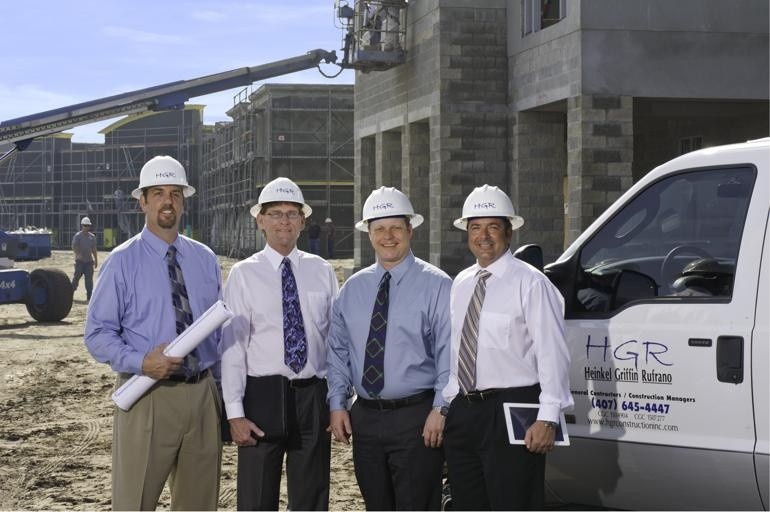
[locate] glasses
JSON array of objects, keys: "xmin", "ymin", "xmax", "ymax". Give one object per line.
[{"xmin": 264, "ymin": 211, "xmax": 300, "ymax": 219}]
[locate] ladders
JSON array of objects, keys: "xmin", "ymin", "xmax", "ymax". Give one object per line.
[
  {"xmin": 4, "ymin": 155, "xmax": 17, "ymax": 182},
  {"xmin": 122, "ymin": 146, "xmax": 136, "ymax": 179}
]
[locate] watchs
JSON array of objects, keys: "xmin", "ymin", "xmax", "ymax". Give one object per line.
[
  {"xmin": 435, "ymin": 404, "xmax": 450, "ymax": 419},
  {"xmin": 543, "ymin": 420, "xmax": 559, "ymax": 429}
]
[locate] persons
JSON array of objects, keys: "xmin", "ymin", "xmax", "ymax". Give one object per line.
[
  {"xmin": 324, "ymin": 186, "xmax": 454, "ymax": 510},
  {"xmin": 442, "ymin": 183, "xmax": 577, "ymax": 510},
  {"xmin": 325, "ymin": 218, "xmax": 336, "ymax": 258},
  {"xmin": 71, "ymin": 216, "xmax": 99, "ymax": 300},
  {"xmin": 219, "ymin": 175, "xmax": 352, "ymax": 510},
  {"xmin": 308, "ymin": 219, "xmax": 322, "ymax": 256},
  {"xmin": 83, "ymin": 154, "xmax": 232, "ymax": 510}
]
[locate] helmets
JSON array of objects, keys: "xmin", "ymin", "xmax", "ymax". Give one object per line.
[
  {"xmin": 250, "ymin": 177, "xmax": 312, "ymax": 219},
  {"xmin": 132, "ymin": 156, "xmax": 196, "ymax": 200},
  {"xmin": 453, "ymin": 184, "xmax": 524, "ymax": 231},
  {"xmin": 355, "ymin": 186, "xmax": 424, "ymax": 232},
  {"xmin": 81, "ymin": 217, "xmax": 92, "ymax": 225}
]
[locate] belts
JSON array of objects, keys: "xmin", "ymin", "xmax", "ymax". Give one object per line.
[
  {"xmin": 168, "ymin": 368, "xmax": 208, "ymax": 384},
  {"xmin": 289, "ymin": 377, "xmax": 319, "ymax": 389},
  {"xmin": 459, "ymin": 384, "xmax": 540, "ymax": 401},
  {"xmin": 357, "ymin": 390, "xmax": 434, "ymax": 409}
]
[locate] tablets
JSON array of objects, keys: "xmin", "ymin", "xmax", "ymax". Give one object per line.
[{"xmin": 503, "ymin": 402, "xmax": 571, "ymax": 446}]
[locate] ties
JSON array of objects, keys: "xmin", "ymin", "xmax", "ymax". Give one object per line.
[
  {"xmin": 282, "ymin": 257, "xmax": 307, "ymax": 374},
  {"xmin": 458, "ymin": 270, "xmax": 492, "ymax": 397},
  {"xmin": 361, "ymin": 273, "xmax": 389, "ymax": 397},
  {"xmin": 166, "ymin": 246, "xmax": 201, "ymax": 377}
]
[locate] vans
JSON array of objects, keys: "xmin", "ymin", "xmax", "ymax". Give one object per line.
[{"xmin": 513, "ymin": 138, "xmax": 769, "ymax": 507}]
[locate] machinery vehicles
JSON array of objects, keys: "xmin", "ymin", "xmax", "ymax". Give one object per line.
[{"xmin": 2, "ymin": 1, "xmax": 409, "ymax": 321}]
